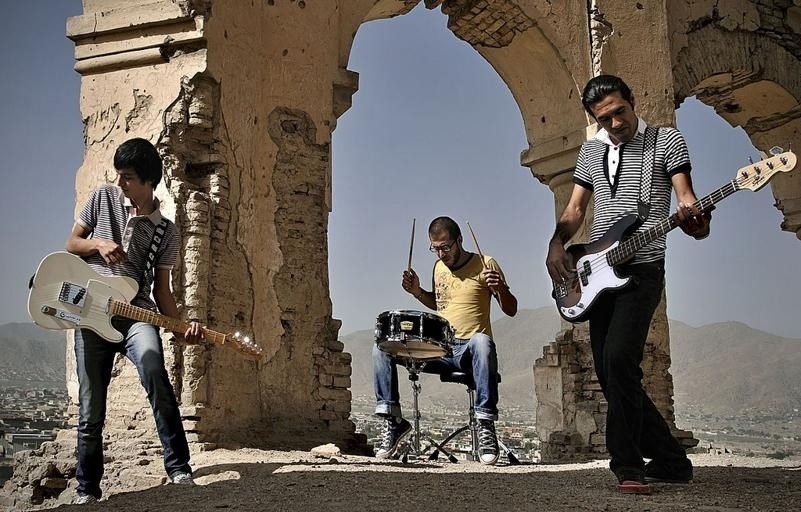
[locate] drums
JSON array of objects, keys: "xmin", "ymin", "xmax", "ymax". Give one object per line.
[{"xmin": 374, "ymin": 310, "xmax": 456, "ymax": 359}]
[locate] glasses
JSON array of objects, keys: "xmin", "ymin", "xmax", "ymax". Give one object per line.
[{"xmin": 429, "ymin": 239, "xmax": 456, "ymax": 252}]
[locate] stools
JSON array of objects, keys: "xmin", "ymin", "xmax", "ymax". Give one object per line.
[{"xmin": 428, "ymin": 371, "xmax": 521, "ymax": 465}]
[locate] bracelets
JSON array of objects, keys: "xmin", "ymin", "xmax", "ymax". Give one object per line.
[
  {"xmin": 416, "ymin": 290, "xmax": 424, "ymax": 300},
  {"xmin": 693, "ymin": 227, "xmax": 710, "ymax": 240}
]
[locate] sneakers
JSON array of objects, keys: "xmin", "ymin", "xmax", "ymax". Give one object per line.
[
  {"xmin": 476, "ymin": 419, "xmax": 500, "ymax": 464},
  {"xmin": 76, "ymin": 493, "xmax": 96, "ymax": 505},
  {"xmin": 173, "ymin": 473, "xmax": 196, "ymax": 486},
  {"xmin": 375, "ymin": 415, "xmax": 413, "ymax": 458},
  {"xmin": 642, "ymin": 461, "xmax": 694, "ymax": 485},
  {"xmin": 618, "ymin": 472, "xmax": 645, "ymax": 486}
]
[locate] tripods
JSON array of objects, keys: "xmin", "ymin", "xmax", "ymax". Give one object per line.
[{"xmin": 392, "ymin": 361, "xmax": 458, "ymax": 464}]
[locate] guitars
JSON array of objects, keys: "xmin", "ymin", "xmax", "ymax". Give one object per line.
[
  {"xmin": 27, "ymin": 250, "xmax": 263, "ymax": 359},
  {"xmin": 551, "ymin": 141, "xmax": 797, "ymax": 323}
]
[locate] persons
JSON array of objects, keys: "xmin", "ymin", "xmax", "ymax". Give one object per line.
[
  {"xmin": 372, "ymin": 216, "xmax": 517, "ymax": 464},
  {"xmin": 545, "ymin": 74, "xmax": 716, "ymax": 494},
  {"xmin": 65, "ymin": 137, "xmax": 207, "ymax": 505}
]
